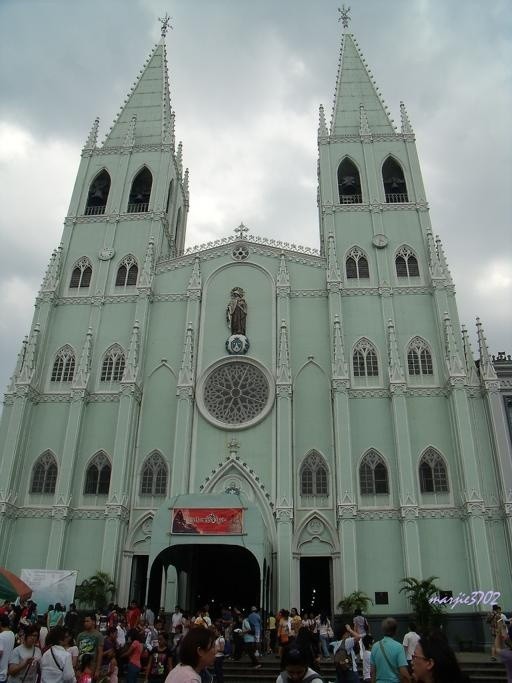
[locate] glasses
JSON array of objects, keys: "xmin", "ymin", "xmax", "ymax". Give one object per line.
[{"xmin": 411, "ymin": 652, "xmax": 432, "ymax": 662}]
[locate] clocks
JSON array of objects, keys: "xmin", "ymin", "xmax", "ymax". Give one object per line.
[
  {"xmin": 372, "ymin": 233, "xmax": 389, "ymax": 249},
  {"xmin": 98, "ymin": 247, "xmax": 115, "ymax": 260}
]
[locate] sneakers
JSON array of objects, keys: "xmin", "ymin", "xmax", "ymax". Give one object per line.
[{"xmin": 254, "ymin": 664, "xmax": 263, "ymax": 669}]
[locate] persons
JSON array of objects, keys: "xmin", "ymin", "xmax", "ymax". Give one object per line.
[
  {"xmin": 225, "ymin": 481, "xmax": 239, "ymax": 494},
  {"xmin": 226, "ymin": 290, "xmax": 248, "ymax": 335},
  {"xmin": 0, "ymin": 593, "xmax": 512, "ymax": 682}
]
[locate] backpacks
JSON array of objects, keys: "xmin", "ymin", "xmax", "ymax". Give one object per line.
[
  {"xmin": 333, "ymin": 639, "xmax": 356, "ymax": 677},
  {"xmin": 219, "ymin": 636, "xmax": 232, "ymax": 655}
]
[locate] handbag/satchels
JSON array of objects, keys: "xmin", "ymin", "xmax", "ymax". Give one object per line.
[
  {"xmin": 364, "ymin": 617, "xmax": 371, "ymax": 633},
  {"xmin": 244, "ymin": 620, "xmax": 256, "ymax": 635}
]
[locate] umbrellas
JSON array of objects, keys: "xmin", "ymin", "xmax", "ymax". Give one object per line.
[{"xmin": 0, "ymin": 565, "xmax": 33, "ymax": 602}]
